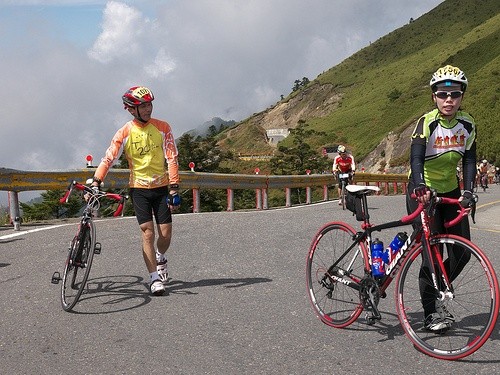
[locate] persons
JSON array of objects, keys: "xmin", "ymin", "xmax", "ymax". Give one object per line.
[
  {"xmin": 494, "ymin": 166, "xmax": 500, "ymax": 185},
  {"xmin": 89, "ymin": 86, "xmax": 180, "ymax": 294},
  {"xmin": 333, "ymin": 145, "xmax": 355, "ymax": 205},
  {"xmin": 405, "ymin": 65, "xmax": 478, "ymax": 331},
  {"xmin": 478, "ymin": 159, "xmax": 490, "ymax": 188}
]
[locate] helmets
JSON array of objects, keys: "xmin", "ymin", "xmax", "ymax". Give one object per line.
[
  {"xmin": 337, "ymin": 145, "xmax": 346, "ymax": 154},
  {"xmin": 496, "ymin": 168, "xmax": 499, "ymax": 171},
  {"xmin": 482, "ymin": 160, "xmax": 487, "ymax": 164},
  {"xmin": 122, "ymin": 87, "xmax": 155, "ymax": 107},
  {"xmin": 429, "ymin": 65, "xmax": 468, "ymax": 90}
]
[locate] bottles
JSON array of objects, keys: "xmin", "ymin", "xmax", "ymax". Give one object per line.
[
  {"xmin": 372, "ymin": 238, "xmax": 384, "ymax": 276},
  {"xmin": 383, "ymin": 232, "xmax": 408, "ymax": 264}
]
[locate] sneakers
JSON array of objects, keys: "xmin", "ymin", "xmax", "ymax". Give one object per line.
[
  {"xmin": 434, "ymin": 300, "xmax": 455, "ymax": 325},
  {"xmin": 149, "ymin": 279, "xmax": 166, "ymax": 295},
  {"xmin": 154, "ymin": 251, "xmax": 169, "ymax": 284},
  {"xmin": 423, "ymin": 312, "xmax": 451, "ymax": 332}
]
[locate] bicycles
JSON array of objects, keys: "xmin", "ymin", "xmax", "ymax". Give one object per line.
[
  {"xmin": 480, "ymin": 171, "xmax": 489, "ymax": 191},
  {"xmin": 303, "ymin": 186, "xmax": 499, "ymax": 361},
  {"xmin": 333, "ymin": 170, "xmax": 352, "ymax": 211},
  {"xmin": 51, "ymin": 181, "xmax": 126, "ymax": 312}
]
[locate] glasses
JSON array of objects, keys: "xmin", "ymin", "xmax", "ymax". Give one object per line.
[{"xmin": 434, "ymin": 91, "xmax": 463, "ymax": 100}]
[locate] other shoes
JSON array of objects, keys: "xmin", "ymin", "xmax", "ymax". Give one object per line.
[{"xmin": 337, "ymin": 196, "xmax": 343, "ymax": 205}]
